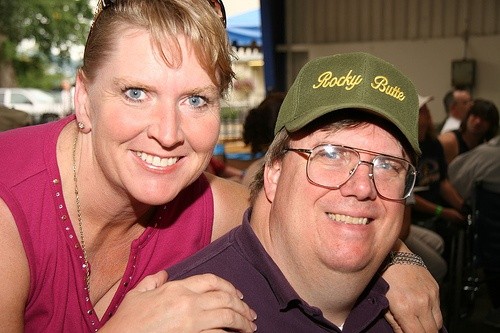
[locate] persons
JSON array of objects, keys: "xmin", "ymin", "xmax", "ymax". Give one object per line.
[
  {"xmin": 242, "ymin": 90, "xmax": 500, "ymax": 333},
  {"xmin": 0, "ymin": 0, "xmax": 443, "ymax": 333},
  {"xmin": 165, "ymin": 52, "xmax": 448, "ymax": 333},
  {"xmin": 204, "ymin": 156, "xmax": 245, "ymax": 183}
]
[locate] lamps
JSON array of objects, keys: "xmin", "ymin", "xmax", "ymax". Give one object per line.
[{"xmin": 451, "ymin": 58, "xmax": 478, "ymax": 88}]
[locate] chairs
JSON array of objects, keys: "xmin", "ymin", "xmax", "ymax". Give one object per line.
[{"xmin": 460, "ymin": 177, "xmax": 500, "ymax": 333}]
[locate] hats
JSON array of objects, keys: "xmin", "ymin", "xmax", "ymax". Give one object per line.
[
  {"xmin": 274, "ymin": 52, "xmax": 422, "ymax": 156},
  {"xmin": 473, "ymin": 99, "xmax": 499, "ymax": 124},
  {"xmin": 417, "ymin": 94, "xmax": 433, "ymax": 109}
]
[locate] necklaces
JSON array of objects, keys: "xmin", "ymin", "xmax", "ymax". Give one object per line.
[{"xmin": 73, "ymin": 128, "xmax": 90, "ymax": 296}]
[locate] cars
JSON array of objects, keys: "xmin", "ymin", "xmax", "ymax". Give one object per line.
[{"xmin": 0, "ymin": 87, "xmax": 67, "ymax": 124}]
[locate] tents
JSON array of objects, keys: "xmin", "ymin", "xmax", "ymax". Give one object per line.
[{"xmin": 226, "ymin": 7, "xmax": 263, "ymax": 48}]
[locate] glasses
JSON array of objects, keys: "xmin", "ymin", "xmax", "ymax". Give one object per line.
[
  {"xmin": 282, "ymin": 143, "xmax": 417, "ymax": 202},
  {"xmin": 90, "ymin": 0, "xmax": 227, "ymax": 35}
]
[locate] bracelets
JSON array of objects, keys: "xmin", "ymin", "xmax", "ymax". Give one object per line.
[
  {"xmin": 379, "ymin": 250, "xmax": 428, "ymax": 273},
  {"xmin": 434, "ymin": 205, "xmax": 443, "ymax": 216}
]
